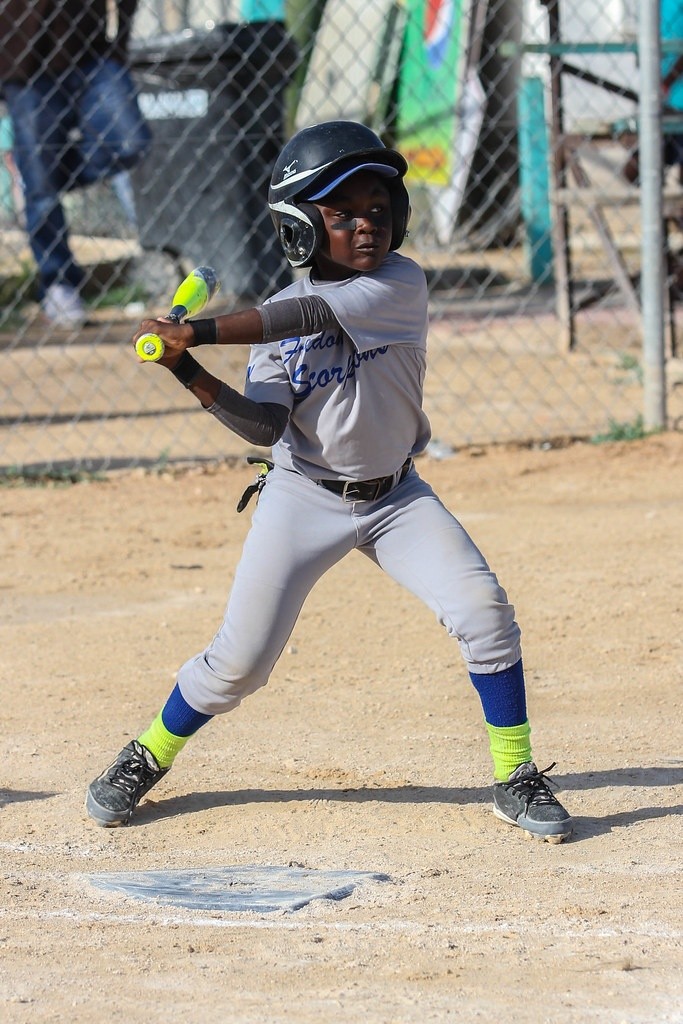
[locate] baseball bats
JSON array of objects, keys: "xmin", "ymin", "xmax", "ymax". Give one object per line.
[{"xmin": 134, "ymin": 265, "xmax": 222, "ymax": 363}]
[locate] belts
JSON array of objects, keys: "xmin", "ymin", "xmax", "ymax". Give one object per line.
[{"xmin": 317, "ymin": 458, "xmax": 415, "ymax": 500}]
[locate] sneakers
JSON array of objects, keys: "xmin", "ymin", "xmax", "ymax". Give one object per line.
[
  {"xmin": 491, "ymin": 760, "xmax": 572, "ymax": 838},
  {"xmin": 85, "ymin": 739, "xmax": 172, "ymax": 828}
]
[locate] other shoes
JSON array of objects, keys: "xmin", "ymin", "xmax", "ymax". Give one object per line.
[{"xmin": 43, "ymin": 283, "xmax": 87, "ymax": 327}]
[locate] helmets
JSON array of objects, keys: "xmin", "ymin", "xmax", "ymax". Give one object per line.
[{"xmin": 266, "ymin": 120, "xmax": 412, "ymax": 268}]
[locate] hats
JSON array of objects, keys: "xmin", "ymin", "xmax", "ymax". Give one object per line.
[{"xmin": 295, "ymin": 160, "xmax": 399, "ymax": 202}]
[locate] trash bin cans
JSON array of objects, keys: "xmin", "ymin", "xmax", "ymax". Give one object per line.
[{"xmin": 122, "ymin": 19, "xmax": 301, "ymax": 304}]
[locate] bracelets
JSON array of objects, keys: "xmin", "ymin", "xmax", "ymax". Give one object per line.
[
  {"xmin": 169, "ymin": 350, "xmax": 205, "ymax": 389},
  {"xmin": 186, "ymin": 318, "xmax": 217, "ymax": 348}
]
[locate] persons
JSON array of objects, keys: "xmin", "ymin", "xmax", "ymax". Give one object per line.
[
  {"xmin": 0, "ymin": 0, "xmax": 153, "ymax": 332},
  {"xmin": 85, "ymin": 121, "xmax": 574, "ymax": 837}
]
